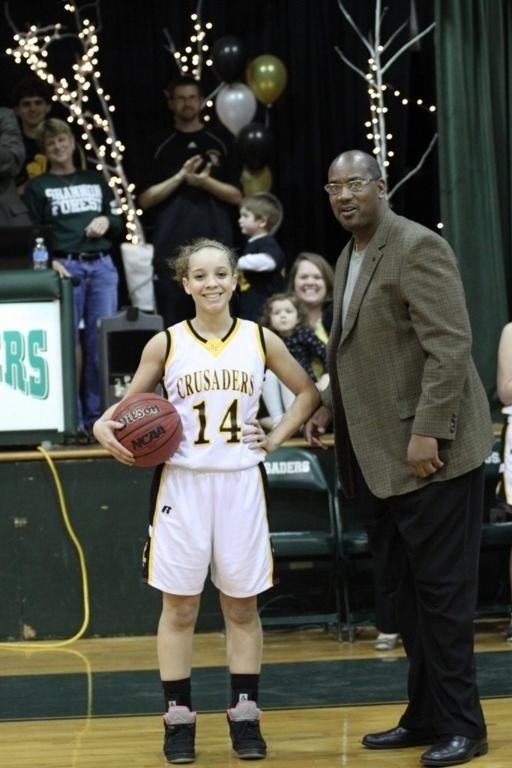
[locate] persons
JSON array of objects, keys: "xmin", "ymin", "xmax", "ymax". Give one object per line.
[
  {"xmin": 12, "ymin": 82, "xmax": 52, "ymax": 198},
  {"xmin": 134, "ymin": 74, "xmax": 247, "ymax": 329},
  {"xmin": 495, "ymin": 323, "xmax": 512, "ymax": 643},
  {"xmin": 0, "ymin": 104, "xmax": 34, "ymax": 272},
  {"xmin": 260, "ymin": 289, "xmax": 333, "ymax": 434},
  {"xmin": 89, "ymin": 236, "xmax": 325, "ymax": 768},
  {"xmin": 18, "ymin": 116, "xmax": 122, "ymax": 446},
  {"xmin": 362, "ymin": 531, "xmax": 403, "ymax": 651},
  {"xmin": 233, "ymin": 191, "xmax": 285, "ymax": 325},
  {"xmin": 288, "ymin": 251, "xmax": 337, "ymax": 385},
  {"xmin": 300, "ymin": 147, "xmax": 496, "ymax": 768}
]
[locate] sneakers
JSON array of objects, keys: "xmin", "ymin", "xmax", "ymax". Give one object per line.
[
  {"xmin": 227, "ymin": 701, "xmax": 267, "ymax": 759},
  {"xmin": 164, "ymin": 706, "xmax": 197, "ymax": 763}
]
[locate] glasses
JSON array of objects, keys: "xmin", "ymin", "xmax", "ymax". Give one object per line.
[{"xmin": 324, "ymin": 177, "xmax": 379, "ymax": 194}]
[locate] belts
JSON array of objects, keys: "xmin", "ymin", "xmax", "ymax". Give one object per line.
[{"xmin": 56, "ymin": 252, "xmax": 109, "ymax": 262}]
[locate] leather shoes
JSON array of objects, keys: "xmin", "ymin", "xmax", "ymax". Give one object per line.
[
  {"xmin": 421, "ymin": 737, "xmax": 488, "ymax": 766},
  {"xmin": 362, "ymin": 726, "xmax": 424, "ymax": 748}
]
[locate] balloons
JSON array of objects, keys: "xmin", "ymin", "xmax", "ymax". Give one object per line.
[
  {"xmin": 229, "ymin": 122, "xmax": 276, "ymax": 177},
  {"xmin": 246, "ymin": 52, "xmax": 289, "ymax": 113},
  {"xmin": 237, "ymin": 162, "xmax": 272, "ymax": 195},
  {"xmin": 215, "ymin": 80, "xmax": 258, "ymax": 145}
]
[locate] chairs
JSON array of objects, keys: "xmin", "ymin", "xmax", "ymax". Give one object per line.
[
  {"xmin": 223, "ymin": 446, "xmax": 346, "ymax": 631},
  {"xmin": 459, "ymin": 438, "xmax": 512, "ymax": 611},
  {"xmin": 332, "ymin": 454, "xmax": 425, "ymax": 618}
]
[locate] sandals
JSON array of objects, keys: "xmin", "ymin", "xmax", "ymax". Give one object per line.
[{"xmin": 374, "ymin": 633, "xmax": 399, "ymax": 651}]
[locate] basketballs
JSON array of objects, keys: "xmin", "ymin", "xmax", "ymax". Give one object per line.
[{"xmin": 111, "ymin": 392, "xmax": 182, "ymax": 468}]
